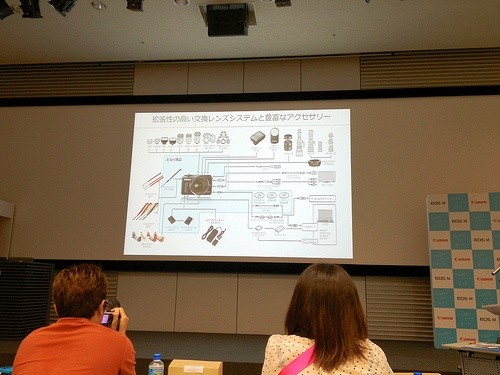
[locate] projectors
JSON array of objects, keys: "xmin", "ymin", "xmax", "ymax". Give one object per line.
[{"xmin": 207, "ymin": 3, "xmax": 249, "ymax": 35}]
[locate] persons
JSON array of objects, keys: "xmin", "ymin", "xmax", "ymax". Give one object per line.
[
  {"xmin": 261, "ymin": 262, "xmax": 396, "ymax": 375},
  {"xmin": 12, "ymin": 264, "xmax": 137, "ymax": 375}
]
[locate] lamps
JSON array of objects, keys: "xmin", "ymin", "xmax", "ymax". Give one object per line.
[{"xmin": 0, "ymin": 0, "xmax": 257, "ymax": 37}]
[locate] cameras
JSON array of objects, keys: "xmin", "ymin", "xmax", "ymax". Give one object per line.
[
  {"xmin": 100, "ymin": 312, "xmax": 121, "ymax": 332},
  {"xmin": 181, "ymin": 175, "xmax": 212, "ymax": 195}
]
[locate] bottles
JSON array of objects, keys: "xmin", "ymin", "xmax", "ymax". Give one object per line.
[{"xmin": 148, "ymin": 353, "xmax": 164, "ymax": 375}]
